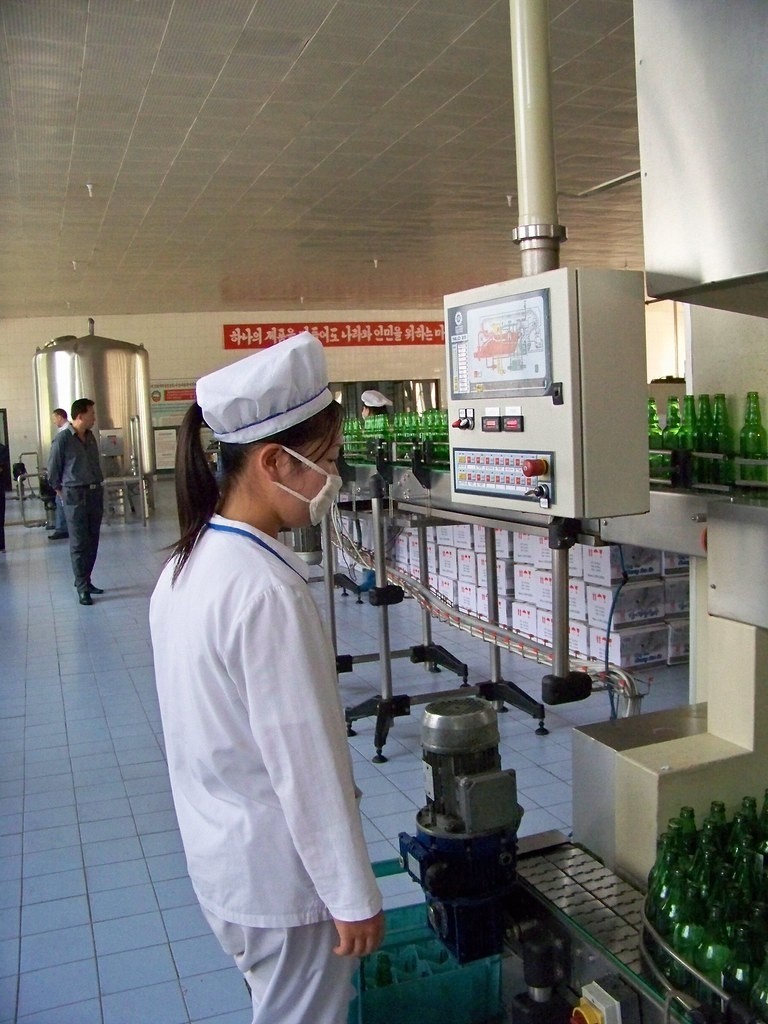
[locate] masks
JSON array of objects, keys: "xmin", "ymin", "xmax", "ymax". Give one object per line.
[{"xmin": 272, "ymin": 444, "xmax": 344, "ymax": 527}]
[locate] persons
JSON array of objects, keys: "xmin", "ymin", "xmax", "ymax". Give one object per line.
[
  {"xmin": 47, "ymin": 408, "xmax": 72, "ymax": 539},
  {"xmin": 46, "ymin": 398, "xmax": 104, "ymax": 606},
  {"xmin": 149, "ymin": 332, "xmax": 385, "ymax": 1024},
  {"xmin": 360, "ymin": 390, "xmax": 393, "ymax": 420}
]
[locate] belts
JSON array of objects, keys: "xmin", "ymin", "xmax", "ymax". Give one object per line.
[{"xmin": 80, "ymin": 484, "xmax": 100, "ymax": 489}]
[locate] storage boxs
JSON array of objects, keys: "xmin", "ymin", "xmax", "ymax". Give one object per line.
[{"xmin": 337, "ymin": 491, "xmax": 689, "ymax": 669}]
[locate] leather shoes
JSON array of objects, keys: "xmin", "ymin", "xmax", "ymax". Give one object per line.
[
  {"xmin": 78, "ymin": 588, "xmax": 93, "ymax": 605},
  {"xmin": 89, "ymin": 583, "xmax": 103, "ymax": 594}
]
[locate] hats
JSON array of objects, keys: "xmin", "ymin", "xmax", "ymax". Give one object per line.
[
  {"xmin": 361, "ymin": 390, "xmax": 393, "ymax": 407},
  {"xmin": 196, "ymin": 330, "xmax": 333, "ymax": 445}
]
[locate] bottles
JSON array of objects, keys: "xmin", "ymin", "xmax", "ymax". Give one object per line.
[
  {"xmin": 663, "ymin": 396, "xmax": 681, "ymax": 491},
  {"xmin": 675, "ymin": 396, "xmax": 696, "ymax": 491},
  {"xmin": 739, "ymin": 392, "xmax": 768, "ymax": 498},
  {"xmin": 647, "ymin": 397, "xmax": 662, "ymax": 487},
  {"xmin": 646, "ymin": 790, "xmax": 768, "ymax": 1013},
  {"xmin": 692, "ymin": 394, "xmax": 717, "ymax": 493},
  {"xmin": 709, "ymin": 394, "xmax": 734, "ymax": 496},
  {"xmin": 342, "ymin": 410, "xmax": 450, "ymax": 467}
]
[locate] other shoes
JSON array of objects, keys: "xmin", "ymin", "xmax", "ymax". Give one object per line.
[
  {"xmin": 2, "ymin": 549, "xmax": 6, "ymax": 553},
  {"xmin": 48, "ymin": 532, "xmax": 69, "ymax": 540}
]
